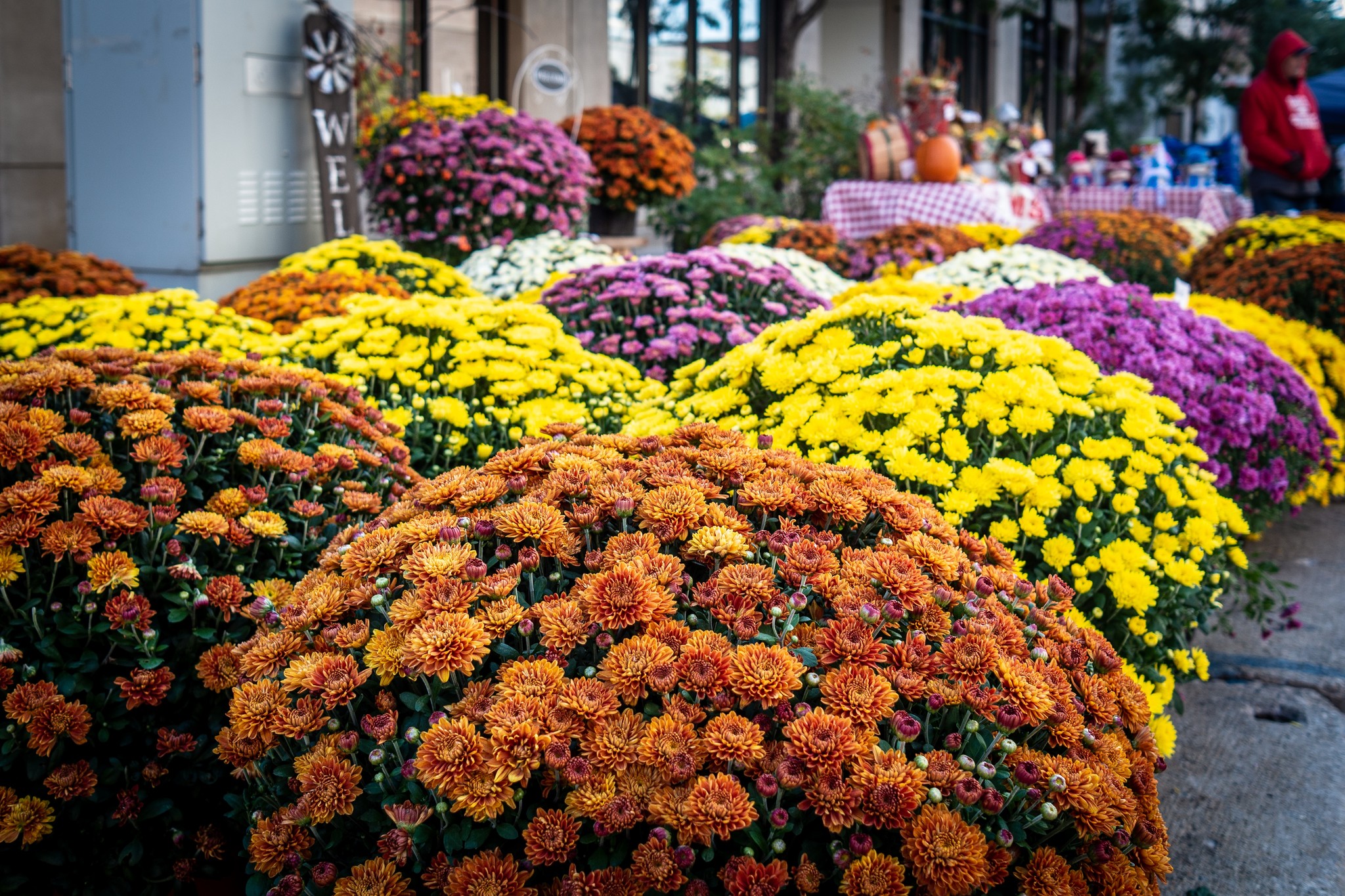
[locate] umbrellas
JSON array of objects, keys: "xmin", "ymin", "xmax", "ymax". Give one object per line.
[{"xmin": 1304, "ymin": 66, "xmax": 1345, "ymax": 111}]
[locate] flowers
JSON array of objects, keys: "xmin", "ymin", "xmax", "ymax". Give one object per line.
[{"xmin": 0, "ymin": 20, "xmax": 1345, "ymax": 896}]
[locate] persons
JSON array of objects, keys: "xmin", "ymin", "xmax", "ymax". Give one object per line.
[
  {"xmin": 1241, "ymin": 29, "xmax": 1331, "ymax": 216},
  {"xmin": 1316, "ymin": 112, "xmax": 1345, "ymax": 214}
]
